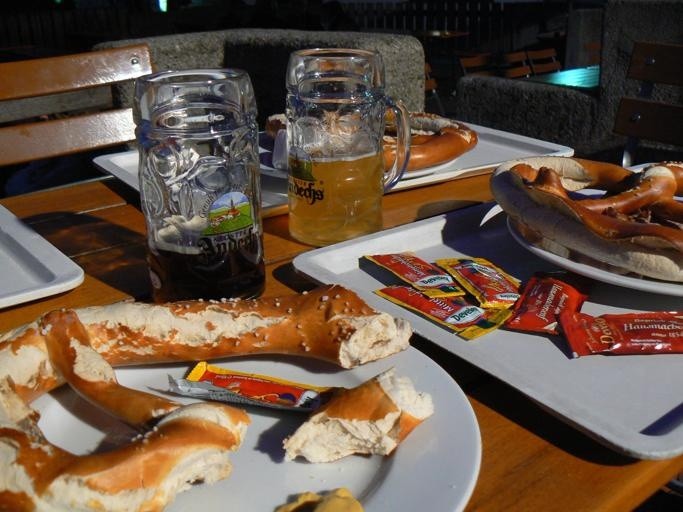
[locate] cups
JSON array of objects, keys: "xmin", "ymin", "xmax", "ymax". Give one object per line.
[
  {"xmin": 133, "ymin": 70, "xmax": 265, "ymax": 299},
  {"xmin": 287, "ymin": 49, "xmax": 410, "ymax": 245}
]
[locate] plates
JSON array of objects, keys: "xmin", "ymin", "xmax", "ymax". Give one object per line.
[
  {"xmin": 260, "ymin": 157, "xmax": 455, "ymax": 185},
  {"xmin": 36, "ymin": 346, "xmax": 484, "ymax": 510},
  {"xmin": 505, "ymin": 207, "xmax": 683, "ymax": 295}
]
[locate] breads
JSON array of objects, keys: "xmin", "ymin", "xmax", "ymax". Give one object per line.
[
  {"xmin": 489, "ymin": 156, "xmax": 683, "ymax": 282},
  {"xmin": 266, "ymin": 104, "xmax": 478, "ymax": 171}
]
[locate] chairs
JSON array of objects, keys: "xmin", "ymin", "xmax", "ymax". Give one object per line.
[
  {"xmin": 425, "ymin": 63, "xmax": 446, "ymax": 115},
  {"xmin": 582, "ymin": 39, "xmax": 602, "ymax": 65},
  {"xmin": 612, "ymin": 41, "xmax": 682, "ymax": 167},
  {"xmin": 458, "ymin": 48, "xmax": 562, "ymax": 78},
  {"xmin": 0, "ymin": 44, "xmax": 154, "ymax": 198}
]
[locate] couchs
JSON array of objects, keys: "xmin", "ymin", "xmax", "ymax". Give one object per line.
[
  {"xmin": 91, "ymin": 27, "xmax": 426, "ymax": 130},
  {"xmin": 456, "ymin": 1, "xmax": 683, "ymax": 157}
]
